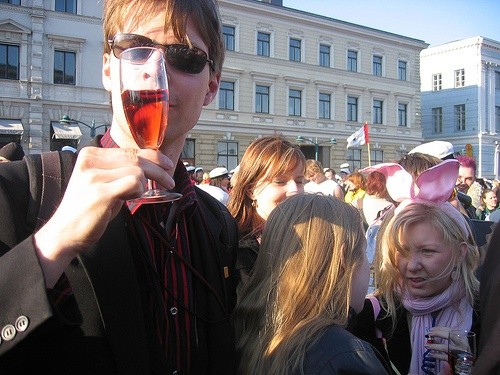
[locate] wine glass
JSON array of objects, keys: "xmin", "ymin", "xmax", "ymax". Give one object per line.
[{"xmin": 118, "ymin": 47, "xmax": 183, "ymax": 204}]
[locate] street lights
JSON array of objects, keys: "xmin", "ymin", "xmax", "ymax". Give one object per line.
[
  {"xmin": 58, "ymin": 114, "xmax": 112, "ymax": 140},
  {"xmin": 295, "ymin": 135, "xmax": 338, "ymax": 162}
]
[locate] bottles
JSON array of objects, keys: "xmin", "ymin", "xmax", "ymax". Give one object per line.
[{"xmin": 457, "ymin": 191, "xmax": 472, "ymax": 210}]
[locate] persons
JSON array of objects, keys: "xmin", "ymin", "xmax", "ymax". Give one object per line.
[
  {"xmin": 183, "ymin": 134, "xmax": 500, "ymax": 375},
  {"xmin": 0, "ymin": 143, "xmax": 25, "ymax": 163},
  {"xmin": 0, "ymin": 0, "xmax": 239, "ymax": 375}
]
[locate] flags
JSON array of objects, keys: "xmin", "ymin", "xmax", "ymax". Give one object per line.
[{"xmin": 346, "ymin": 122, "xmax": 370, "ymax": 149}]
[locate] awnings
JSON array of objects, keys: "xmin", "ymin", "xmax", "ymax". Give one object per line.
[
  {"xmin": 0, "ymin": 119, "xmax": 24, "ymax": 135},
  {"xmin": 50, "ymin": 122, "xmax": 83, "ymax": 139}
]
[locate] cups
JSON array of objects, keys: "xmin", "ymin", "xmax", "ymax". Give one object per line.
[{"xmin": 448, "ymin": 330, "xmax": 477, "ymax": 375}]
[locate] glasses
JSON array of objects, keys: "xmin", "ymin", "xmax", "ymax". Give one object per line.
[{"xmin": 107, "ymin": 33, "xmax": 216, "ymax": 74}]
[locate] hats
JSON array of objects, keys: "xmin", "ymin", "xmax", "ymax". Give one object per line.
[
  {"xmin": 408, "ymin": 140, "xmax": 454, "ymax": 160},
  {"xmin": 0, "ymin": 142, "xmax": 25, "ymax": 161},
  {"xmin": 187, "ymin": 166, "xmax": 195, "ymax": 173},
  {"xmin": 62, "ymin": 146, "xmax": 76, "ymax": 154},
  {"xmin": 209, "ymin": 167, "xmax": 229, "ymax": 179},
  {"xmin": 195, "ymin": 167, "xmax": 203, "ymax": 173}
]
